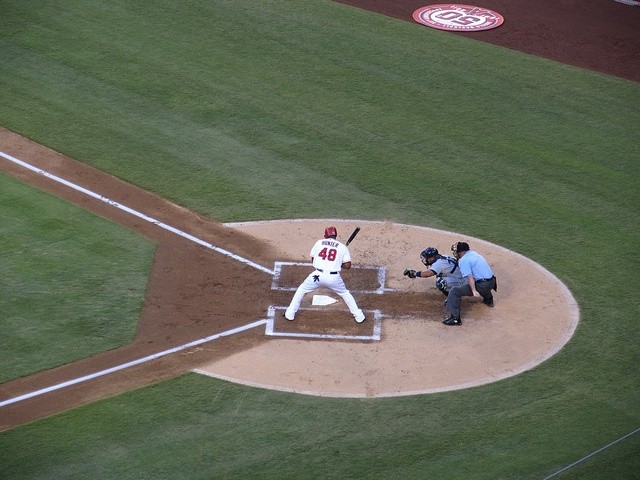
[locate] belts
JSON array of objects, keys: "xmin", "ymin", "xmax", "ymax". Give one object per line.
[{"xmin": 475, "ymin": 275, "xmax": 495, "ymax": 283}]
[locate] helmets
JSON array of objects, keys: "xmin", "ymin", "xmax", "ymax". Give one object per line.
[
  {"xmin": 324, "ymin": 225, "xmax": 338, "ymax": 238},
  {"xmin": 419, "ymin": 247, "xmax": 439, "ymax": 266},
  {"xmin": 451, "ymin": 242, "xmax": 469, "ymax": 252}
]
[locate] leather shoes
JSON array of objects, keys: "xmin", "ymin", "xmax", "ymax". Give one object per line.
[
  {"xmin": 484, "ymin": 298, "xmax": 493, "ymax": 307},
  {"xmin": 442, "ymin": 313, "xmax": 462, "ymax": 326}
]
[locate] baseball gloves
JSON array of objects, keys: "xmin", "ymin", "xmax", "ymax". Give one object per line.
[{"xmin": 404, "ymin": 269, "xmax": 415, "ymax": 279}]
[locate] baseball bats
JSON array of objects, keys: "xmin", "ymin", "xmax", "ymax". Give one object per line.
[{"xmin": 345, "ymin": 227, "xmax": 360, "ymax": 246}]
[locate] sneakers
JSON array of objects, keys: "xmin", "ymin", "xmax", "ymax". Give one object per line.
[
  {"xmin": 355, "ymin": 315, "xmax": 368, "ymax": 322},
  {"xmin": 283, "ymin": 313, "xmax": 293, "ymax": 321}
]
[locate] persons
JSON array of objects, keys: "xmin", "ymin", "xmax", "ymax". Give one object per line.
[
  {"xmin": 442, "ymin": 242, "xmax": 497, "ymax": 326},
  {"xmin": 281, "ymin": 226, "xmax": 367, "ymax": 324},
  {"xmin": 403, "ymin": 247, "xmax": 466, "ymax": 305}
]
